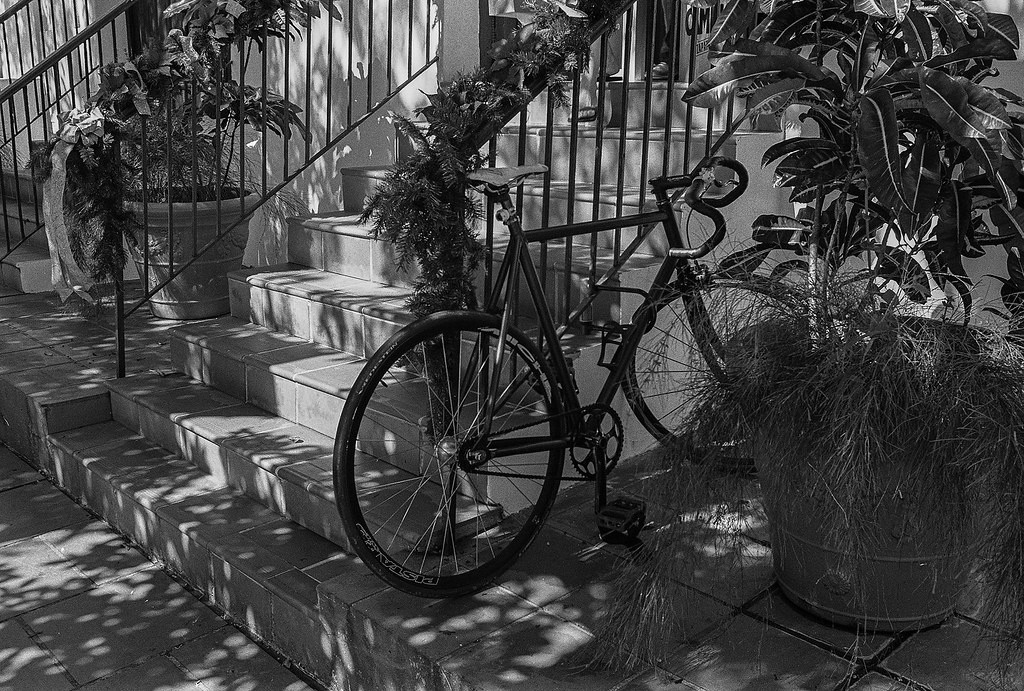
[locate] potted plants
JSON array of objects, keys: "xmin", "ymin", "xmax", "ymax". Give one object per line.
[
  {"xmin": 577, "ymin": 0, "xmax": 1024, "ymax": 691},
  {"xmin": 83, "ymin": 1, "xmax": 343, "ymax": 321}
]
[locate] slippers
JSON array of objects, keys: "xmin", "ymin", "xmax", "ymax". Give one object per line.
[
  {"xmin": 596, "ymin": 70, "xmax": 623, "ymax": 81},
  {"xmin": 567, "ymin": 105, "xmax": 597, "ymax": 122}
]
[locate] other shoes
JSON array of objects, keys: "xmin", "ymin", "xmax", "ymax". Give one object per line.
[{"xmin": 643, "ymin": 61, "xmax": 669, "ymax": 80}]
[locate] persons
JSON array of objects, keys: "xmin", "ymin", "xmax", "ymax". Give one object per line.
[
  {"xmin": 567, "ymin": 14, "xmax": 623, "ymax": 123},
  {"xmin": 643, "ymin": 0, "xmax": 680, "ymax": 82}
]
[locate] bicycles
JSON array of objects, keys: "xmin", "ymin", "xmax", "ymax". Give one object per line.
[{"xmin": 331, "ymin": 153, "xmax": 838, "ymax": 601}]
